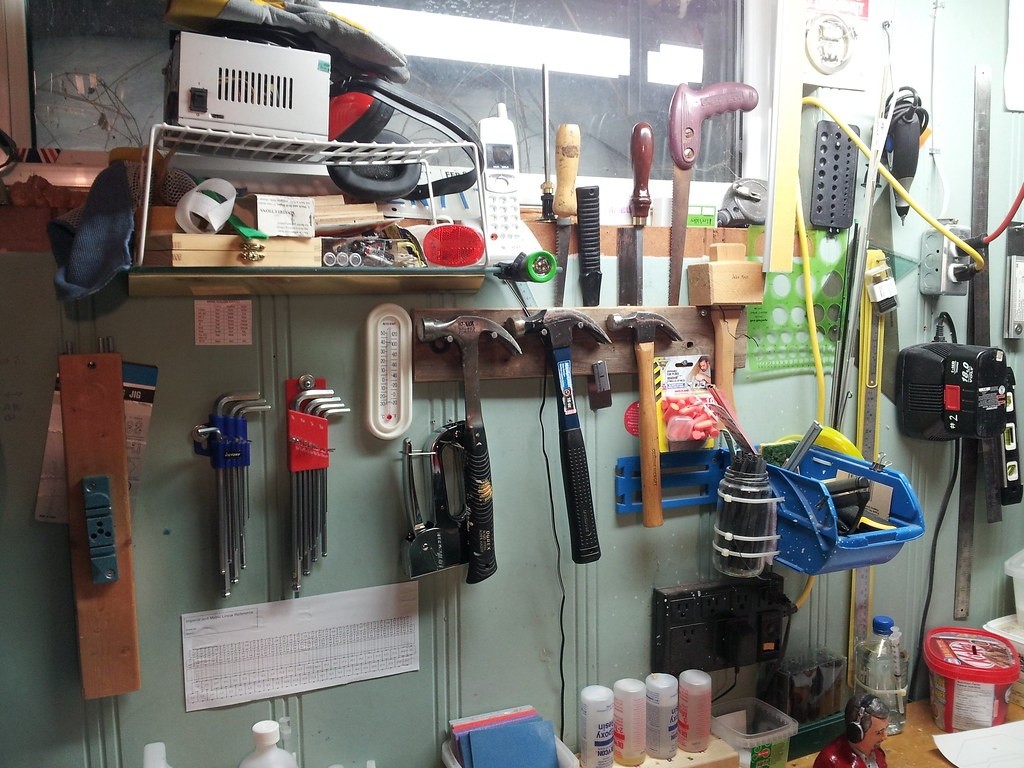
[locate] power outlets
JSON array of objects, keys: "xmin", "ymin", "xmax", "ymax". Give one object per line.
[
  {"xmin": 653, "ymin": 570, "xmax": 785, "ymax": 680},
  {"xmin": 919, "ymin": 221, "xmax": 972, "ymax": 297}
]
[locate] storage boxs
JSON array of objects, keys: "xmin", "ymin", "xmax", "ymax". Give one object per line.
[
  {"xmin": 735, "ymin": 442, "xmax": 925, "ymax": 578},
  {"xmin": 441, "ymin": 644, "xmax": 850, "ymax": 768}
]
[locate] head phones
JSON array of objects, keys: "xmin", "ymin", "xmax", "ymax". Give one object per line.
[{"xmin": 327, "ymin": 72, "xmax": 484, "ymax": 203}]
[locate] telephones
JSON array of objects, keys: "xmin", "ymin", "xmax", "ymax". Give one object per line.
[{"xmin": 477, "ymin": 101, "xmax": 523, "ymax": 264}]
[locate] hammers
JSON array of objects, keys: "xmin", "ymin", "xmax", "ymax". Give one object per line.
[
  {"xmin": 605, "ymin": 312, "xmax": 683, "ymax": 528},
  {"xmin": 416, "ymin": 315, "xmax": 522, "ymax": 584},
  {"xmin": 506, "ymin": 308, "xmax": 614, "ymax": 565}
]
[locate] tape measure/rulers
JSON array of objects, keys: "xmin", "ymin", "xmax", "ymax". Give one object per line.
[
  {"xmin": 951, "ymin": 62, "xmax": 1003, "ymax": 619},
  {"xmin": 848, "ymin": 250, "xmax": 886, "ymax": 684}
]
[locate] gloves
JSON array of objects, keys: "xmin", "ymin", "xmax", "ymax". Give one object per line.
[{"xmin": 167, "ymin": 0, "xmax": 409, "ymax": 85}]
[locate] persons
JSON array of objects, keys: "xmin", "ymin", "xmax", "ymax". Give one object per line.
[
  {"xmin": 695, "ymin": 358, "xmax": 712, "ymax": 385},
  {"xmin": 813, "ymin": 692, "xmax": 890, "ymax": 768}
]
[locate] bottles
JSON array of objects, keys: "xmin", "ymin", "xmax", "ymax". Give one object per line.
[
  {"xmin": 580, "ymin": 669, "xmax": 712, "ymax": 768},
  {"xmin": 856, "ymin": 616, "xmax": 908, "ymax": 736},
  {"xmin": 144, "ymin": 742, "xmax": 172, "ymax": 768},
  {"xmin": 712, "ymin": 466, "xmax": 772, "ymax": 577},
  {"xmin": 239, "ymin": 720, "xmax": 297, "ymax": 768}
]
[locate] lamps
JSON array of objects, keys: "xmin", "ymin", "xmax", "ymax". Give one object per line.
[{"xmin": 895, "ymin": 339, "xmax": 1008, "ymax": 440}]
